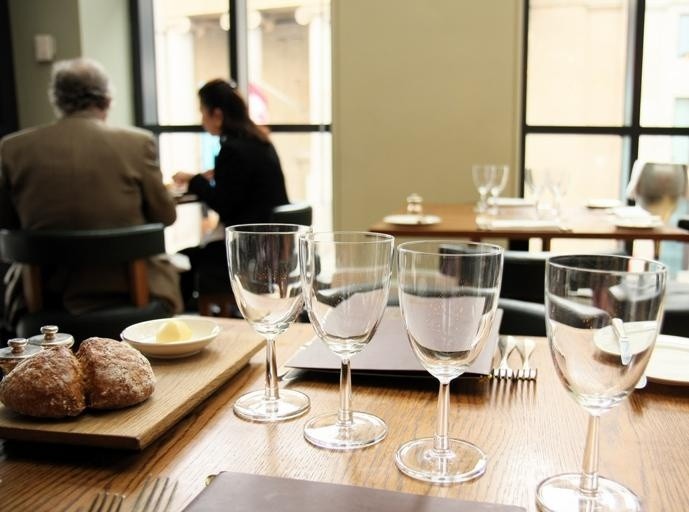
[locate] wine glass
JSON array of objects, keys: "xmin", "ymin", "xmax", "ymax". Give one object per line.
[
  {"xmin": 486, "ymin": 165, "xmax": 509, "ymax": 214},
  {"xmin": 536, "ymin": 253, "xmax": 667, "ymax": 512},
  {"xmin": 472, "ymin": 165, "xmax": 496, "ymax": 213},
  {"xmin": 394, "ymin": 240, "xmax": 506, "ymax": 485},
  {"xmin": 300, "ymin": 229, "xmax": 395, "ymax": 450},
  {"xmin": 224, "ymin": 222, "xmax": 312, "ymax": 423}
]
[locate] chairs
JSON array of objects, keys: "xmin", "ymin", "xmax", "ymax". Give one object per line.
[
  {"xmin": 0, "ymin": 222, "xmax": 166, "ymax": 337},
  {"xmin": 196, "ymin": 204, "xmax": 312, "ymax": 318}
]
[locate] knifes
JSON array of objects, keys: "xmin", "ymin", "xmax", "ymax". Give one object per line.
[
  {"xmin": 631, "ymin": 372, "xmax": 649, "ymax": 389},
  {"xmin": 612, "ymin": 318, "xmax": 634, "ymax": 364}
]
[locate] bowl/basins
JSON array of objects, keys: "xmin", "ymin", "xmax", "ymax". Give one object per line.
[{"xmin": 120, "ymin": 317, "xmax": 221, "ymax": 359}]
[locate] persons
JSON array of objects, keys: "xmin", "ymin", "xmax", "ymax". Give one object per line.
[
  {"xmin": 171, "ymin": 79, "xmax": 289, "ymax": 314},
  {"xmin": 0, "ymin": 57, "xmax": 185, "ymax": 325}
]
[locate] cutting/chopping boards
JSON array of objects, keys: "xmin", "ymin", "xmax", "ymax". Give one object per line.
[{"xmin": 0, "ymin": 309, "xmax": 268, "ymax": 458}]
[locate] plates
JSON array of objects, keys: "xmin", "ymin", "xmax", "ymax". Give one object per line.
[
  {"xmin": 627, "ymin": 334, "xmax": 689, "ymax": 386},
  {"xmin": 586, "ymin": 196, "xmax": 658, "ymax": 230},
  {"xmin": 594, "ymin": 321, "xmax": 658, "ymax": 356},
  {"xmin": 488, "ymin": 197, "xmax": 535, "ymax": 211},
  {"xmin": 384, "ymin": 214, "xmax": 441, "ymax": 226}
]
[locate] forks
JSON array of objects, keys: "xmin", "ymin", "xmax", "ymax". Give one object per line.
[
  {"xmin": 490, "ymin": 335, "xmax": 517, "ymax": 382},
  {"xmin": 512, "ymin": 339, "xmax": 538, "ymax": 381}
]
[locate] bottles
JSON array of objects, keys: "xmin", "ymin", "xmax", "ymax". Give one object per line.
[
  {"xmin": 27, "ymin": 323, "xmax": 76, "ymax": 354},
  {"xmin": 406, "ymin": 193, "xmax": 424, "ymax": 216},
  {"xmin": 0, "ymin": 336, "xmax": 45, "ymax": 377}
]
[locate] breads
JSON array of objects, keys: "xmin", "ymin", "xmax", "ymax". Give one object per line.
[{"xmin": 0, "ymin": 336, "xmax": 155, "ymax": 420}]
